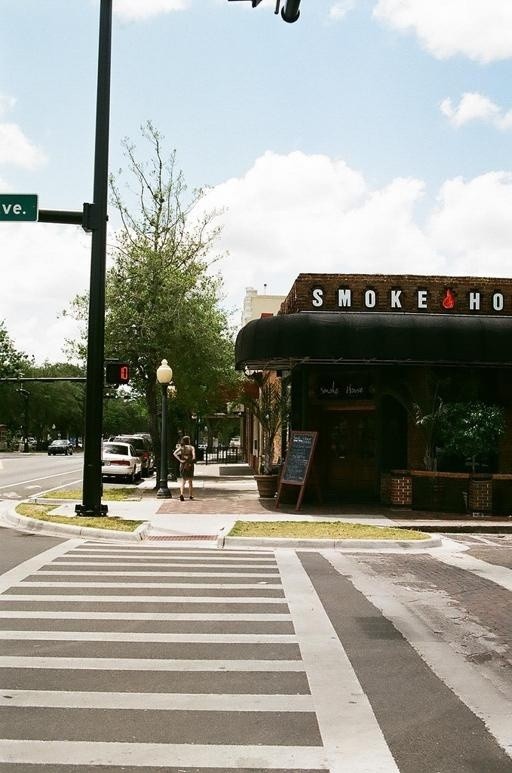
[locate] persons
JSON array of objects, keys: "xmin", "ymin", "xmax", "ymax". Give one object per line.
[{"xmin": 173, "ymin": 435, "xmax": 196, "ymax": 502}]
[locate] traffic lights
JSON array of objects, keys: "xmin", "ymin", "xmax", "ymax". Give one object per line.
[{"xmin": 106, "ymin": 363, "xmax": 130, "ymax": 384}]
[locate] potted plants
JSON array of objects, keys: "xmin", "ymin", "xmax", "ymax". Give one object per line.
[
  {"xmin": 438, "ymin": 398, "xmax": 507, "ymax": 510},
  {"xmin": 381, "ymin": 373, "xmax": 467, "ymax": 510},
  {"xmin": 229, "ymin": 384, "xmax": 297, "ymax": 499}
]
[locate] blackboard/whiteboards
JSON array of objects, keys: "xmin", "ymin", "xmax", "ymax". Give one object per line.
[{"xmin": 281, "ymin": 430, "xmax": 316, "ymax": 482}]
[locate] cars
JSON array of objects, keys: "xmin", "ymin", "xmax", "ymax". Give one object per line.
[{"xmin": 47, "ymin": 439, "xmax": 74, "ymax": 456}]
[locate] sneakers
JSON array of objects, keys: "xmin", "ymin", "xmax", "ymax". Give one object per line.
[{"xmin": 180, "ymin": 495, "xmax": 194, "ymax": 501}]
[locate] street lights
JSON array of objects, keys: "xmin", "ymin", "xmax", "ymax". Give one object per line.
[
  {"xmin": 101, "ymin": 432, "xmax": 155, "ymax": 483},
  {"xmin": 156, "ymin": 357, "xmax": 173, "ymax": 499}
]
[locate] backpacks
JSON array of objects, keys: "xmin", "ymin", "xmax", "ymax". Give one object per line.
[{"xmin": 182, "ymin": 446, "xmax": 193, "ymax": 471}]
